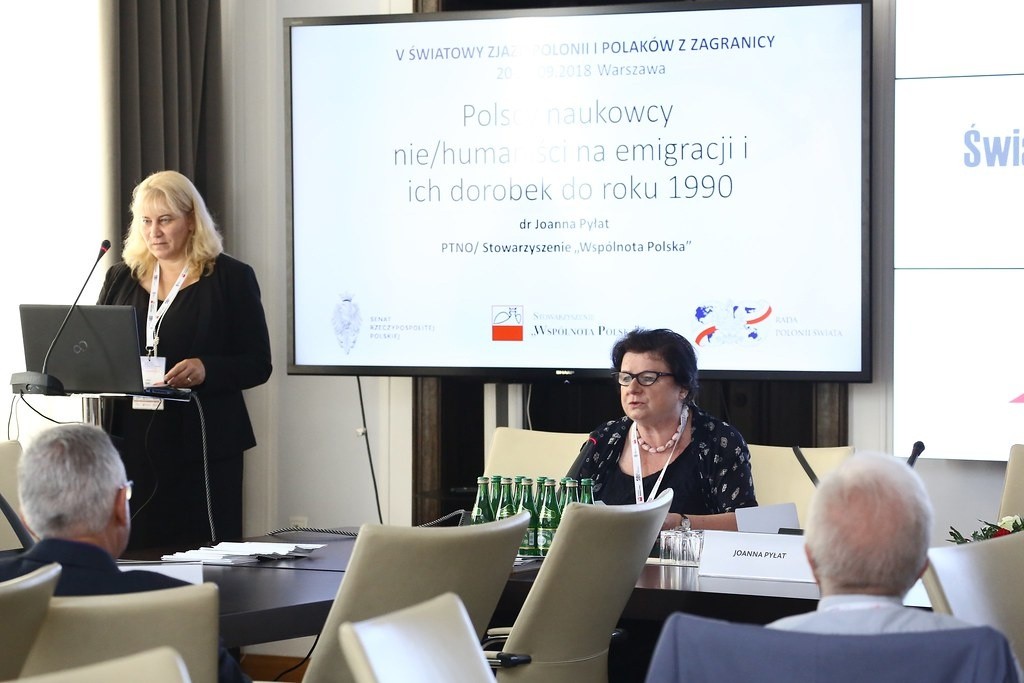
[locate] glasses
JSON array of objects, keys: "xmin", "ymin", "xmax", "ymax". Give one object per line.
[
  {"xmin": 119, "ymin": 479, "xmax": 134, "ymax": 501},
  {"xmin": 611, "ymin": 370, "xmax": 675, "ymax": 386}
]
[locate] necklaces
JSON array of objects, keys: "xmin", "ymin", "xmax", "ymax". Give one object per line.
[{"xmin": 636, "ymin": 424, "xmax": 681, "ymax": 452}]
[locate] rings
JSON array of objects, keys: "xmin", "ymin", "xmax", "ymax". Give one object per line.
[{"xmin": 187, "ymin": 378, "xmax": 192, "ymax": 382}]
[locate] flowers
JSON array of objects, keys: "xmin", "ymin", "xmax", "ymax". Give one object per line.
[{"xmin": 945, "ymin": 514, "xmax": 1024, "ymax": 545}]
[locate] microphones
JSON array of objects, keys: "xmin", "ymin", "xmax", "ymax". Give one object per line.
[{"xmin": 11, "ymin": 240, "xmax": 111, "ymax": 395}]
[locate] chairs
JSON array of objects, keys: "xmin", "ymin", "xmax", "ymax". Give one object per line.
[
  {"xmin": 0, "ymin": 439, "xmax": 32, "ymax": 552},
  {"xmin": 0, "ymin": 563, "xmax": 219, "ymax": 683},
  {"xmin": 301, "ymin": 487, "xmax": 673, "ymax": 683},
  {"xmin": 121, "ymin": 525, "xmax": 934, "ymax": 650},
  {"xmin": 746, "ymin": 443, "xmax": 855, "ymax": 528},
  {"xmin": 470, "ymin": 427, "xmax": 590, "ymax": 517},
  {"xmin": 643, "ymin": 613, "xmax": 1024, "ymax": 683},
  {"xmin": 920, "ymin": 443, "xmax": 1024, "ymax": 683}
]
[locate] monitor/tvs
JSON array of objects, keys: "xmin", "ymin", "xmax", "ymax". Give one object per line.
[{"xmin": 279, "ymin": 1, "xmax": 877, "ymax": 382}]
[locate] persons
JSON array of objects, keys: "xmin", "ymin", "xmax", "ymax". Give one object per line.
[
  {"xmin": 96, "ymin": 171, "xmax": 273, "ymax": 548},
  {"xmin": 765, "ymin": 449, "xmax": 1024, "ymax": 682},
  {"xmin": 0, "ymin": 425, "xmax": 251, "ymax": 683},
  {"xmin": 580, "ymin": 329, "xmax": 758, "ymax": 530}
]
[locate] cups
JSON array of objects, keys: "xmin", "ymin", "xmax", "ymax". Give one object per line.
[
  {"xmin": 680, "ymin": 533, "xmax": 703, "ymax": 564},
  {"xmin": 659, "ymin": 531, "xmax": 683, "ymax": 564}
]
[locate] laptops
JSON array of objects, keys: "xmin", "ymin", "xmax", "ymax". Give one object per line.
[{"xmin": 19, "ymin": 304, "xmax": 193, "ymax": 399}]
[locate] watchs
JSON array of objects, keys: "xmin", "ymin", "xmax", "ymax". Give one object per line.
[{"xmin": 679, "ymin": 513, "xmax": 690, "ymax": 529}]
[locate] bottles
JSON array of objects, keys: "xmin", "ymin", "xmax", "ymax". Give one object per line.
[
  {"xmin": 559, "ymin": 477, "xmax": 572, "ymax": 513},
  {"xmin": 490, "ymin": 476, "xmax": 501, "ymax": 521},
  {"xmin": 518, "ymin": 478, "xmax": 538, "ymax": 555},
  {"xmin": 538, "ymin": 479, "xmax": 562, "ymax": 557},
  {"xmin": 580, "ymin": 478, "xmax": 594, "ymax": 505},
  {"xmin": 496, "ymin": 478, "xmax": 517, "ymax": 520},
  {"xmin": 514, "ymin": 476, "xmax": 525, "ymax": 515},
  {"xmin": 533, "ymin": 477, "xmax": 548, "ymax": 514},
  {"xmin": 564, "ymin": 480, "xmax": 579, "ymax": 508},
  {"xmin": 471, "ymin": 477, "xmax": 495, "ymax": 525}
]
